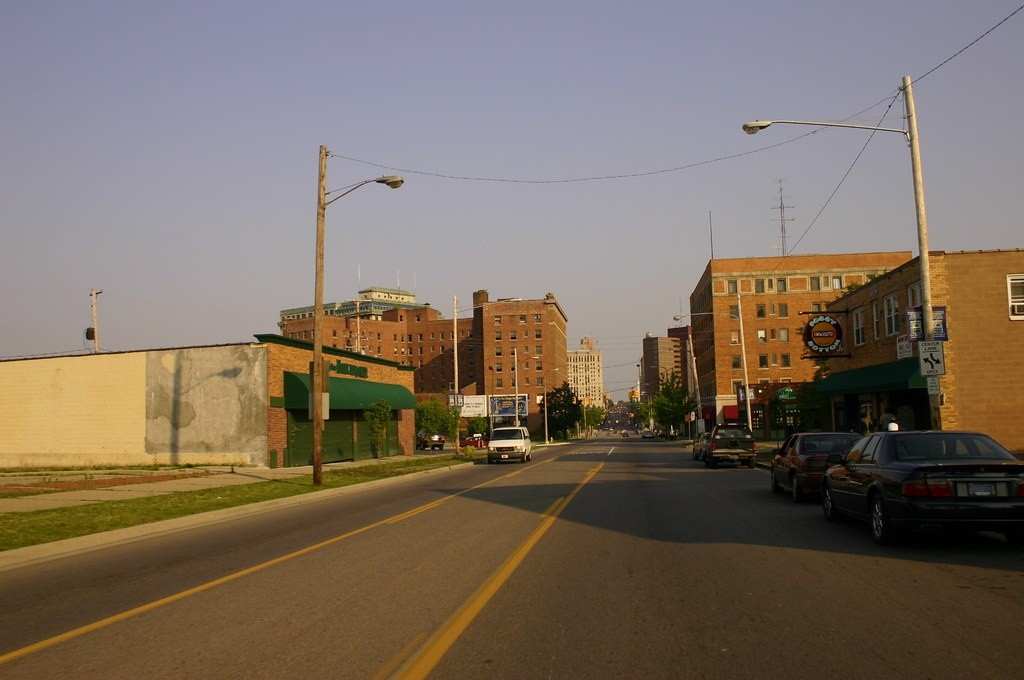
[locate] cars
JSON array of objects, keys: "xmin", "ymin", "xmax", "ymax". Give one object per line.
[
  {"xmin": 623, "ymin": 432, "xmax": 629, "ymax": 437},
  {"xmin": 417, "ymin": 431, "xmax": 445, "ymax": 451},
  {"xmin": 460, "ymin": 435, "xmax": 487, "ymax": 448},
  {"xmin": 822, "ymin": 430, "xmax": 1024, "ymax": 543},
  {"xmin": 605, "ymin": 427, "xmax": 608, "ymax": 430},
  {"xmin": 769, "ymin": 431, "xmax": 866, "ymax": 500},
  {"xmin": 609, "ymin": 428, "xmax": 613, "ymax": 431},
  {"xmin": 692, "ymin": 432, "xmax": 711, "ymax": 460},
  {"xmin": 642, "ymin": 431, "xmax": 653, "ymax": 438}
]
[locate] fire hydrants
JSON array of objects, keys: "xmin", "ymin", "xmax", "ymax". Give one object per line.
[{"xmin": 550, "ymin": 437, "xmax": 553, "ymax": 443}]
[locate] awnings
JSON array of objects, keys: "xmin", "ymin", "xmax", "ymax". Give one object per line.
[
  {"xmin": 818, "ymin": 357, "xmax": 927, "ymax": 397},
  {"xmin": 284, "ymin": 370, "xmax": 417, "ymax": 410}
]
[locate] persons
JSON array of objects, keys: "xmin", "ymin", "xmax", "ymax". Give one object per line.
[
  {"xmin": 879, "ymin": 408, "xmax": 896, "ymax": 432},
  {"xmin": 483, "ymin": 428, "xmax": 488, "ymax": 437}
]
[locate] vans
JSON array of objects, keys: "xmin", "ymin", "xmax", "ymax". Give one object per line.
[{"xmin": 488, "ymin": 427, "xmax": 533, "ymax": 463}]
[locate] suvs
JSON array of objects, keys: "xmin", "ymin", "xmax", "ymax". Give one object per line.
[{"xmin": 705, "ymin": 422, "xmax": 757, "ymax": 469}]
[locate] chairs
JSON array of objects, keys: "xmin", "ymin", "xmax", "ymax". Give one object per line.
[{"xmin": 804, "ymin": 442, "xmax": 816, "ymax": 450}]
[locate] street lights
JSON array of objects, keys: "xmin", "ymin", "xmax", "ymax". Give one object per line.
[
  {"xmin": 673, "ymin": 312, "xmax": 753, "ymax": 432},
  {"xmin": 311, "ymin": 175, "xmax": 405, "ymax": 483},
  {"xmin": 650, "ymin": 366, "xmax": 668, "ymax": 385},
  {"xmin": 744, "ymin": 119, "xmax": 943, "ymax": 431},
  {"xmin": 452, "ymin": 297, "xmax": 522, "ymax": 454},
  {"xmin": 543, "ymin": 368, "xmax": 560, "ymax": 443}
]
